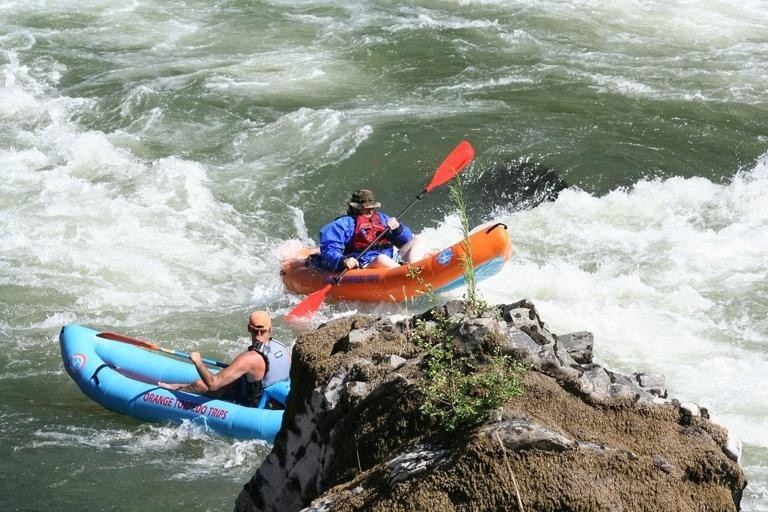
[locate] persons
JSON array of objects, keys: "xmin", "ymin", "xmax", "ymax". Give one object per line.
[
  {"xmin": 318, "ymin": 188, "xmax": 414, "ymax": 270},
  {"xmin": 157, "ymin": 310, "xmax": 292, "ymax": 408}
]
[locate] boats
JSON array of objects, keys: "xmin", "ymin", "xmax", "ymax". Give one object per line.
[
  {"xmin": 280, "ymin": 223, "xmax": 511, "ymax": 303},
  {"xmin": 59, "ymin": 324, "xmax": 290, "ymax": 445}
]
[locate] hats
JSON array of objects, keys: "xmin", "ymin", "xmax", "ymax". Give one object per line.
[
  {"xmin": 246, "ymin": 310, "xmax": 273, "ymax": 332},
  {"xmin": 347, "ymin": 189, "xmax": 382, "ymax": 210}
]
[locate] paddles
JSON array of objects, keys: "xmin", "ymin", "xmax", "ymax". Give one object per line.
[
  {"xmin": 282, "ymin": 141, "xmax": 475, "ymax": 317},
  {"xmin": 96, "ymin": 332, "xmax": 230, "ymax": 369}
]
